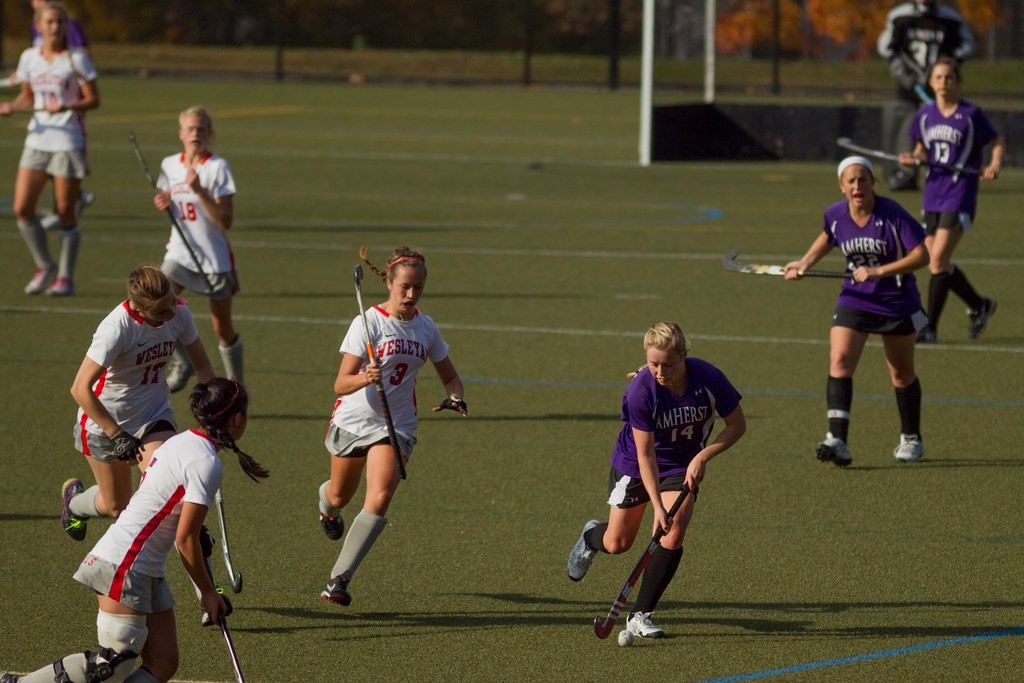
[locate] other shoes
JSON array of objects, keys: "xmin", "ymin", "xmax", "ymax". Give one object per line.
[
  {"xmin": 0, "ymin": 669, "xmax": 23, "ymax": 683},
  {"xmin": 888, "ymin": 173, "xmax": 919, "ymax": 191}
]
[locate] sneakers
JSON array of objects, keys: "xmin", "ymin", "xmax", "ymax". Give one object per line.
[
  {"xmin": 626, "ymin": 611, "xmax": 665, "ymax": 639},
  {"xmin": 965, "ymin": 296, "xmax": 1000, "ymax": 338},
  {"xmin": 915, "ymin": 329, "xmax": 938, "ymax": 343},
  {"xmin": 320, "ymin": 574, "xmax": 353, "ymax": 606},
  {"xmin": 23, "ymin": 266, "xmax": 58, "ymax": 294},
  {"xmin": 45, "ymin": 277, "xmax": 75, "ymax": 296},
  {"xmin": 814, "ymin": 431, "xmax": 852, "ymax": 467},
  {"xmin": 166, "ymin": 360, "xmax": 194, "ymax": 392},
  {"xmin": 318, "ymin": 479, "xmax": 343, "ymax": 540},
  {"xmin": 893, "ymin": 433, "xmax": 925, "ymax": 461},
  {"xmin": 61, "ymin": 478, "xmax": 91, "ymax": 540},
  {"xmin": 566, "ymin": 520, "xmax": 601, "ymax": 581}
]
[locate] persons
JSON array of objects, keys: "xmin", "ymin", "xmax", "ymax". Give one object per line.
[
  {"xmin": 567, "ymin": 320, "xmax": 747, "ymax": 637},
  {"xmin": 0, "ymin": 0, "xmax": 103, "ymax": 295},
  {"xmin": 152, "ymin": 104, "xmax": 246, "ymax": 392},
  {"xmin": 779, "ymin": 155, "xmax": 930, "ymax": 468},
  {"xmin": 898, "ymin": 54, "xmax": 999, "ymax": 346},
  {"xmin": 875, "ymin": 0, "xmax": 982, "ymax": 194},
  {"xmin": 0, "ymin": 376, "xmax": 270, "ymax": 683},
  {"xmin": 58, "ymin": 267, "xmax": 216, "ymax": 542},
  {"xmin": 317, "ymin": 245, "xmax": 469, "ymax": 606}
]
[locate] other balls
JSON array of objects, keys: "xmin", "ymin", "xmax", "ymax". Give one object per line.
[{"xmin": 617, "ymin": 631, "xmax": 634, "ymax": 647}]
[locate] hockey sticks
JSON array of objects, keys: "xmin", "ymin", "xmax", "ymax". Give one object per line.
[
  {"xmin": 217, "ymin": 618, "xmax": 245, "ymax": 683},
  {"xmin": 353, "ymin": 265, "xmax": 409, "ymax": 481},
  {"xmin": 215, "ymin": 489, "xmax": 244, "ymax": 593},
  {"xmin": 722, "ymin": 254, "xmax": 901, "ymax": 283},
  {"xmin": 593, "ymin": 483, "xmax": 690, "ymax": 641},
  {"xmin": 836, "ymin": 137, "xmax": 987, "ymax": 175},
  {"xmin": 127, "ymin": 131, "xmax": 215, "ymax": 293}
]
